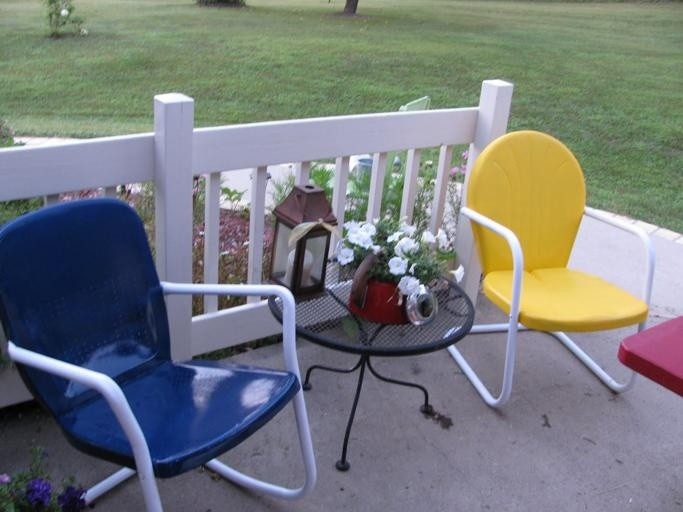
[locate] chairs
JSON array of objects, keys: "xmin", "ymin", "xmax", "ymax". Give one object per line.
[
  {"xmin": 1, "ymin": 195, "xmax": 319, "ymax": 509},
  {"xmin": 448, "ymin": 127, "xmax": 649, "ymax": 414}
]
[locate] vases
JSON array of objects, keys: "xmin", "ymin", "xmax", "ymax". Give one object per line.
[{"xmin": 347, "ymin": 277, "xmax": 410, "ymax": 322}]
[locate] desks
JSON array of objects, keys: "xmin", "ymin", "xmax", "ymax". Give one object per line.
[{"xmin": 267, "ymin": 275, "xmax": 474, "ymax": 473}]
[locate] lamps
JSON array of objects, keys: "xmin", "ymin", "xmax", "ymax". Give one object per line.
[{"xmin": 264, "ymin": 182, "xmax": 338, "ymax": 303}]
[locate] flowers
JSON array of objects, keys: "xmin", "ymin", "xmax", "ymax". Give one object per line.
[{"xmin": 343, "ymin": 215, "xmax": 466, "ymax": 300}]
